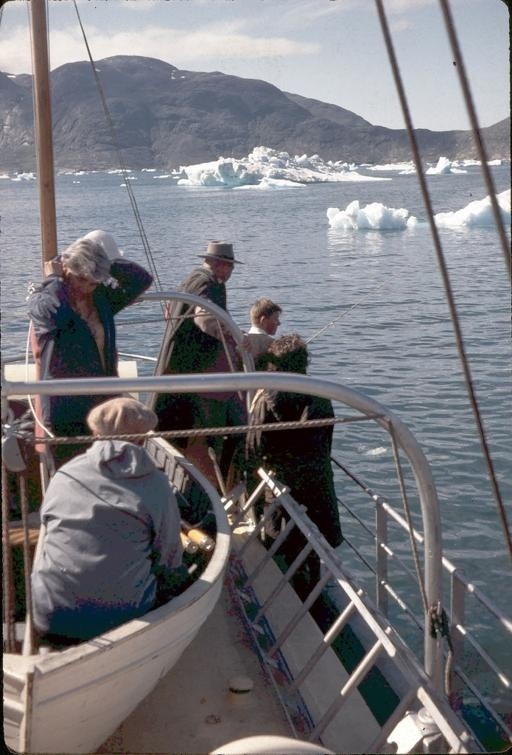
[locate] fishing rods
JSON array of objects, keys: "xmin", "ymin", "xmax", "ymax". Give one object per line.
[{"xmin": 305, "ymin": 272, "xmax": 401, "ymax": 346}]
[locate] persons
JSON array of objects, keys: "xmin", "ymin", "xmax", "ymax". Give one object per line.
[
  {"xmin": 27, "ymin": 238, "xmax": 154, "ymax": 486},
  {"xmin": 143, "ymin": 238, "xmax": 245, "ymax": 464},
  {"xmin": 247, "ymin": 333, "xmax": 343, "ymax": 625},
  {"xmin": 240, "ymin": 296, "xmax": 283, "ymax": 394},
  {"xmin": 28, "ymin": 396, "xmax": 193, "ymax": 646}
]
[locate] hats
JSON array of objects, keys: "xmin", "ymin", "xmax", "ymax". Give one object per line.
[
  {"xmin": 195, "ymin": 238, "xmax": 246, "ymax": 267},
  {"xmin": 84, "ymin": 396, "xmax": 161, "ymax": 444}
]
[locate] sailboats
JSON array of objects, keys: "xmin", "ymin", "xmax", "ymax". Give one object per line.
[{"xmin": 0, "ymin": 3, "xmax": 512, "ymax": 755}]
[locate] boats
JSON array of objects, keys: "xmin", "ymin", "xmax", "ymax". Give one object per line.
[{"xmin": 2, "ymin": 392, "xmax": 236, "ymax": 754}]
